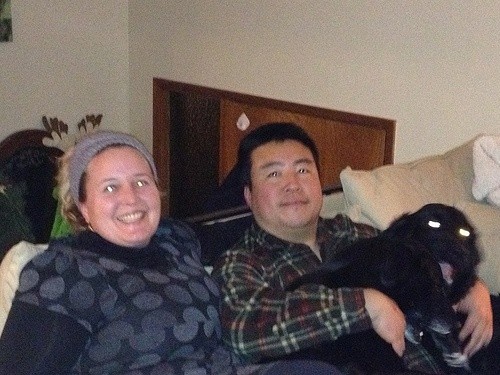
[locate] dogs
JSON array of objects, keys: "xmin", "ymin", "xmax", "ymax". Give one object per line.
[{"xmin": 284, "ymin": 203, "xmax": 483, "ymax": 375}]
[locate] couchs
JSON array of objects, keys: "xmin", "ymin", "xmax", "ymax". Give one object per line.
[{"xmin": 183, "ymin": 187, "xmax": 344, "ymax": 275}]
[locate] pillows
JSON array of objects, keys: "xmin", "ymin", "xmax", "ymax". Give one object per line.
[
  {"xmin": 0, "ymin": 240, "xmax": 49, "ymax": 338},
  {"xmin": 340, "ymin": 135, "xmax": 500, "ymax": 296}
]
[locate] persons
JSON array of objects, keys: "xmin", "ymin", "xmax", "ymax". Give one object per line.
[
  {"xmin": 211, "ymin": 121, "xmax": 492, "ymax": 375},
  {"xmin": 0, "ymin": 130, "xmax": 337, "ymax": 375}
]
